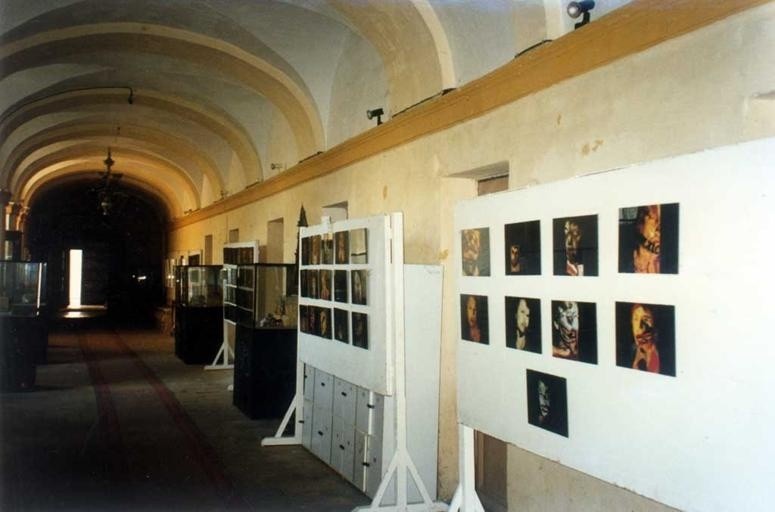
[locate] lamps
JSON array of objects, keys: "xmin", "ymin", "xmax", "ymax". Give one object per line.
[
  {"xmin": 566, "ymin": 2, "xmax": 594, "ymax": 28},
  {"xmin": 367, "ymin": 107, "xmax": 381, "ymax": 126}
]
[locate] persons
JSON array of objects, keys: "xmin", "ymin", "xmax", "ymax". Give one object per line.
[
  {"xmin": 534, "ymin": 378, "xmax": 551, "ymax": 421},
  {"xmin": 551, "ymin": 301, "xmax": 587, "ymax": 361},
  {"xmin": 460, "ymin": 294, "xmax": 488, "ymax": 345},
  {"xmin": 505, "ymin": 240, "xmax": 529, "ymax": 275},
  {"xmin": 625, "ymin": 303, "xmax": 673, "ymax": 375},
  {"xmin": 505, "ymin": 297, "xmax": 542, "ymax": 354},
  {"xmin": 461, "ymin": 227, "xmax": 480, "ymax": 275},
  {"xmin": 631, "ymin": 204, "xmax": 660, "ymax": 274},
  {"xmin": 553, "ymin": 219, "xmax": 598, "ymax": 276}
]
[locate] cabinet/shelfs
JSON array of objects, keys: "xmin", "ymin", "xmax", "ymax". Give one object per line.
[
  {"xmin": 229, "ymin": 262, "xmax": 296, "ymax": 421},
  {"xmin": 168, "ymin": 263, "xmax": 224, "ymax": 365},
  {"xmin": 1, "ymin": 261, "xmax": 44, "ymax": 394},
  {"xmin": 299, "ymin": 266, "xmax": 442, "ymax": 505}
]
